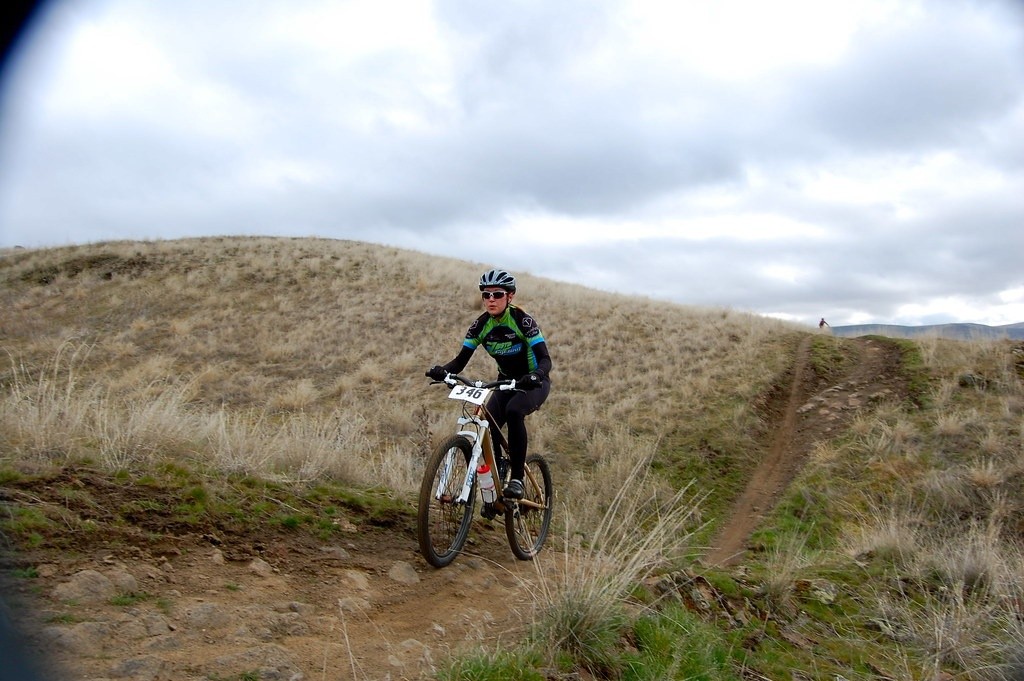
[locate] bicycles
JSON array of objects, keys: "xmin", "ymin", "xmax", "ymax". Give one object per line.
[{"xmin": 417, "ymin": 370, "xmax": 553, "ymax": 569}]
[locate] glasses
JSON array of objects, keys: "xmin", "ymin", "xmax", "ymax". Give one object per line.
[{"xmin": 482, "ymin": 291, "xmax": 512, "ymax": 300}]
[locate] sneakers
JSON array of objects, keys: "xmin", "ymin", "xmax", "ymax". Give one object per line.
[
  {"xmin": 503, "ymin": 480, "xmax": 525, "ymax": 500},
  {"xmin": 481, "ymin": 500, "xmax": 495, "ymax": 518}
]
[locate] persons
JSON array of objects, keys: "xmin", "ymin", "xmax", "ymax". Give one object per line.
[
  {"xmin": 431, "ymin": 268, "xmax": 552, "ymax": 520},
  {"xmin": 819, "ymin": 318, "xmax": 828, "ymax": 328}
]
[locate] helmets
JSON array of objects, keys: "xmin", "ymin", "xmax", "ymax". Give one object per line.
[{"xmin": 478, "ymin": 269, "xmax": 515, "ymax": 292}]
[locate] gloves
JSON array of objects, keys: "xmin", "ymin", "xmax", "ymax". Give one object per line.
[
  {"xmin": 430, "ymin": 364, "xmax": 448, "ymax": 383},
  {"xmin": 519, "ymin": 373, "xmax": 539, "ymax": 391}
]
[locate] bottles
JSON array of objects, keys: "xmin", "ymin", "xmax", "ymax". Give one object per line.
[{"xmin": 477, "ymin": 465, "xmax": 498, "ymax": 503}]
